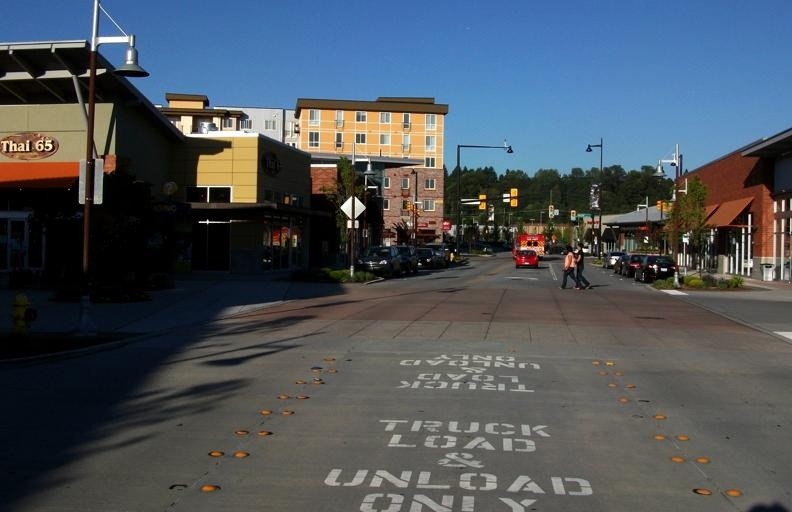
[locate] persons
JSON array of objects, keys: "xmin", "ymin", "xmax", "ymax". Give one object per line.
[
  {"xmin": 573, "ymin": 242, "xmax": 592, "ymax": 290},
  {"xmin": 559, "ymin": 245, "xmax": 582, "ymax": 290}
]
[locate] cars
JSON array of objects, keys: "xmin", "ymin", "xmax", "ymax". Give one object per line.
[
  {"xmin": 358, "ymin": 241, "xmax": 455, "ymax": 278},
  {"xmin": 513, "ymin": 249, "xmax": 542, "ymax": 269},
  {"xmin": 600, "ymin": 250, "xmax": 683, "ymax": 284}
]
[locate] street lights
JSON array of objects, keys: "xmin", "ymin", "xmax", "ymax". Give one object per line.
[
  {"xmin": 653, "ymin": 141, "xmax": 680, "ymax": 279},
  {"xmin": 78, "ymin": 0, "xmax": 153, "ymax": 300},
  {"xmin": 410, "ymin": 167, "xmax": 419, "ymax": 248},
  {"xmin": 638, "ymin": 195, "xmax": 650, "ymax": 255},
  {"xmin": 455, "ymin": 140, "xmax": 515, "ymax": 259},
  {"xmin": 586, "ymin": 138, "xmax": 602, "ymax": 260},
  {"xmin": 348, "ymin": 141, "xmax": 377, "ymax": 277}
]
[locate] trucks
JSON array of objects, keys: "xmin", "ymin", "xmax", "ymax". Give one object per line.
[{"xmin": 576, "ymin": 243, "xmax": 596, "ymax": 255}]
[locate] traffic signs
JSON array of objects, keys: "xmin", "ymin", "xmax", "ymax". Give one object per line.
[{"xmin": 577, "ymin": 213, "xmax": 592, "ymax": 217}]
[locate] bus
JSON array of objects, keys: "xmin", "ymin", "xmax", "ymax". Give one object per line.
[{"xmin": 512, "ymin": 233, "xmax": 547, "ymax": 259}]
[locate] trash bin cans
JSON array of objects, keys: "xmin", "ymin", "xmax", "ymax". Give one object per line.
[{"xmin": 760, "ymin": 263, "xmax": 774, "ymax": 282}]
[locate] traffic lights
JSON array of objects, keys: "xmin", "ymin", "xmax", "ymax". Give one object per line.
[
  {"xmin": 657, "ymin": 201, "xmax": 662, "ymax": 210},
  {"xmin": 548, "ymin": 205, "xmax": 554, "ymax": 219},
  {"xmin": 571, "ymin": 210, "xmax": 576, "ymax": 221}
]
